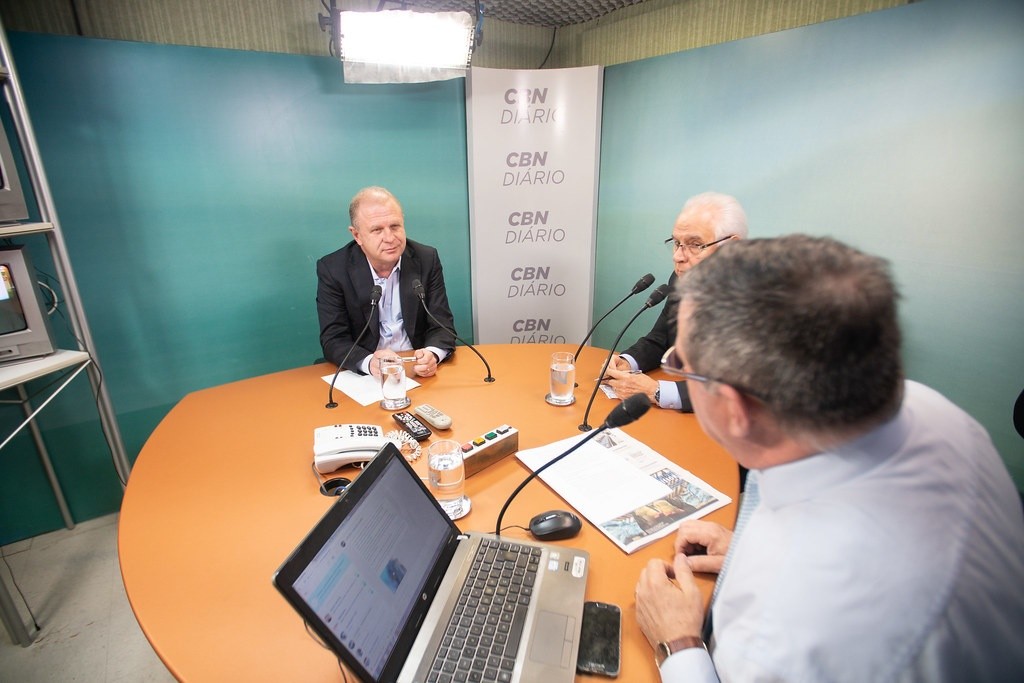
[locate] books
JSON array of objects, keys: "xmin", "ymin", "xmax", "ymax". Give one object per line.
[{"xmin": 515, "ymin": 427, "xmax": 732, "ymax": 554}]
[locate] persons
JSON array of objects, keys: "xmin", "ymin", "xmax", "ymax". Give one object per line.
[
  {"xmin": 316, "ymin": 186, "xmax": 458, "ymax": 378},
  {"xmin": 635, "ymin": 233, "xmax": 1023, "ymax": 683},
  {"xmin": 601, "ymin": 191, "xmax": 749, "ymax": 413}
]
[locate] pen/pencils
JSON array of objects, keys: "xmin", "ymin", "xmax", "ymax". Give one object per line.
[
  {"xmin": 595, "ymin": 370, "xmax": 642, "ymax": 381},
  {"xmin": 399, "ymin": 357, "xmax": 421, "ymax": 362}
]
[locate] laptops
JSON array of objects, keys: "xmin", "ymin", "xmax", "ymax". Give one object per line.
[{"xmin": 272, "ymin": 442, "xmax": 590, "ymax": 683}]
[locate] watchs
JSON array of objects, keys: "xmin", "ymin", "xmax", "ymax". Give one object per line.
[
  {"xmin": 654, "ymin": 636, "xmax": 708, "ymax": 668},
  {"xmin": 654, "ymin": 388, "xmax": 660, "ymax": 406}
]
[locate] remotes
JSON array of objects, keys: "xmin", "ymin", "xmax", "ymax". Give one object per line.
[
  {"xmin": 414, "ymin": 404, "xmax": 452, "ymax": 429},
  {"xmin": 392, "ymin": 411, "xmax": 432, "ymax": 442}
]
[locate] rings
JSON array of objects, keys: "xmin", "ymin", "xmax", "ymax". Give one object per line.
[
  {"xmin": 427, "ymin": 368, "xmax": 430, "ymax": 372},
  {"xmin": 615, "ymin": 387, "xmax": 616, "ymax": 391}
]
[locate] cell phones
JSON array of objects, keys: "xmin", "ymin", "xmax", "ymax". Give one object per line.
[{"xmin": 576, "ymin": 602, "xmax": 622, "ymax": 677}]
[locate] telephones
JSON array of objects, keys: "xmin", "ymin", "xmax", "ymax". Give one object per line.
[{"xmin": 314, "ymin": 424, "xmax": 402, "ymax": 474}]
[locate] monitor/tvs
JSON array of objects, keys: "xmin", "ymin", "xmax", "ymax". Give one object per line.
[
  {"xmin": 0, "ymin": 119, "xmax": 28, "ymax": 227},
  {"xmin": 0, "ymin": 249, "xmax": 57, "ymax": 363}
]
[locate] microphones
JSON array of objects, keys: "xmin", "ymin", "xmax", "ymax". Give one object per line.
[
  {"xmin": 575, "ymin": 274, "xmax": 655, "ymax": 361},
  {"xmin": 496, "ymin": 392, "xmax": 653, "ymax": 537},
  {"xmin": 326, "ymin": 286, "xmax": 382, "ymax": 408},
  {"xmin": 412, "ymin": 279, "xmax": 495, "ymax": 382},
  {"xmin": 578, "ymin": 285, "xmax": 671, "ymax": 433}
]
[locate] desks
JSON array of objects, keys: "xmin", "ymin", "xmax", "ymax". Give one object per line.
[
  {"xmin": 118, "ymin": 343, "xmax": 742, "ymax": 683},
  {"xmin": 0, "ymin": 350, "xmax": 93, "ymax": 647}
]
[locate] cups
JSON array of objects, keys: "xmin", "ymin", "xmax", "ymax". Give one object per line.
[
  {"xmin": 427, "ymin": 439, "xmax": 466, "ymax": 517},
  {"xmin": 550, "ymin": 352, "xmax": 576, "ymax": 404},
  {"xmin": 379, "ymin": 356, "xmax": 407, "ymax": 409}
]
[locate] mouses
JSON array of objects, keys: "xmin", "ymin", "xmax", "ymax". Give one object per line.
[{"xmin": 529, "ymin": 510, "xmax": 582, "ymax": 541}]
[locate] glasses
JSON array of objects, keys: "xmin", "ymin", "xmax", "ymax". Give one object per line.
[
  {"xmin": 660, "ymin": 346, "xmax": 772, "ymax": 409},
  {"xmin": 665, "ymin": 235, "xmax": 738, "ymax": 258}
]
